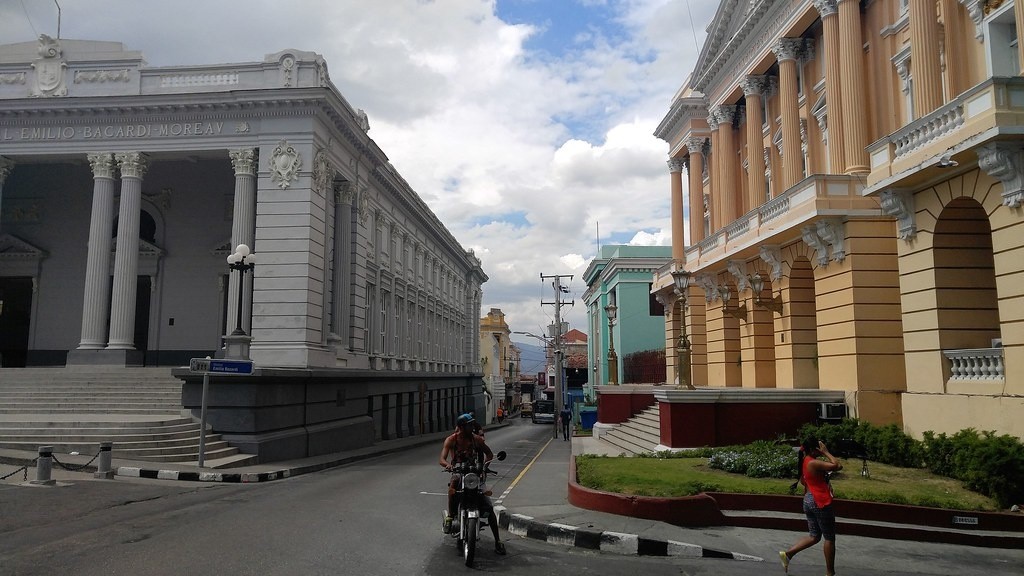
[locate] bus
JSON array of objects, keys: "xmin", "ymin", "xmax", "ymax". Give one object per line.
[
  {"xmin": 519, "ymin": 403, "xmax": 532, "ymax": 418},
  {"xmin": 530, "ymin": 398, "xmax": 554, "ymax": 424}
]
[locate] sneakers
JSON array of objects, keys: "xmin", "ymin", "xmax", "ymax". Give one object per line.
[
  {"xmin": 494, "ymin": 540, "xmax": 506, "ymax": 555},
  {"xmin": 443, "ymin": 515, "xmax": 453, "ymax": 534}
]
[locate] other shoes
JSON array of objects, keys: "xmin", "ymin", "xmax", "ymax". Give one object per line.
[{"xmin": 779, "ymin": 551, "xmax": 790, "ymax": 573}]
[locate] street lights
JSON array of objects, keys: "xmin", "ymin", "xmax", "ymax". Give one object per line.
[
  {"xmin": 527, "ymin": 333, "xmax": 566, "ymax": 432},
  {"xmin": 603, "ymin": 301, "xmax": 620, "ymax": 386},
  {"xmin": 223, "ymin": 244, "xmax": 257, "ymax": 336},
  {"xmin": 669, "ymin": 264, "xmax": 696, "ymax": 390},
  {"xmin": 513, "ymin": 330, "xmax": 561, "ymax": 439}
]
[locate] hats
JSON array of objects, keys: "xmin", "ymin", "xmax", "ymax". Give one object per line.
[{"xmin": 456, "ymin": 414, "xmax": 475, "ymax": 425}]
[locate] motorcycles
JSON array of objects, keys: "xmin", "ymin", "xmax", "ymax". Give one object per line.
[{"xmin": 440, "ymin": 450, "xmax": 508, "ymax": 568}]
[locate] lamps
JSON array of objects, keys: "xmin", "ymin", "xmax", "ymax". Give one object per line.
[
  {"xmin": 746, "ymin": 272, "xmax": 783, "ymax": 317},
  {"xmin": 717, "ymin": 282, "xmax": 747, "ymax": 322}
]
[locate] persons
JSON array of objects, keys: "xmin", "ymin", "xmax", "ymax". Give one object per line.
[
  {"xmin": 440, "ymin": 412, "xmax": 507, "ymax": 554},
  {"xmin": 497, "ymin": 407, "xmax": 508, "ymax": 424},
  {"xmin": 560, "ymin": 404, "xmax": 572, "ymax": 441},
  {"xmin": 778, "ymin": 437, "xmax": 842, "ymax": 576}
]
